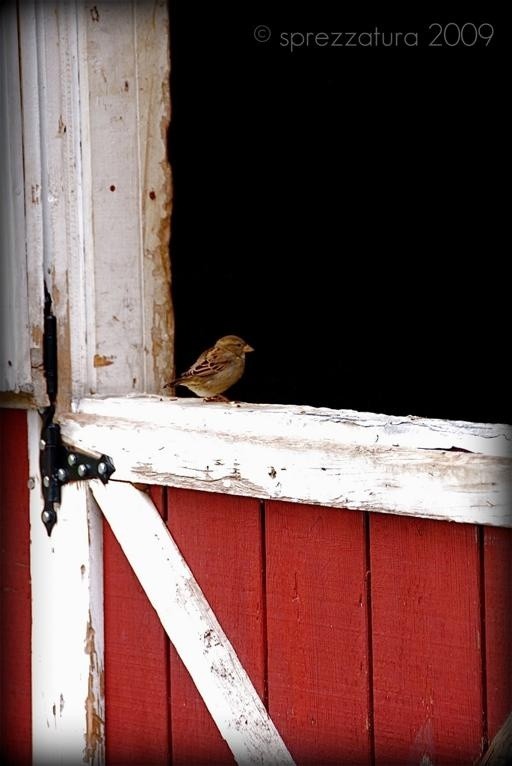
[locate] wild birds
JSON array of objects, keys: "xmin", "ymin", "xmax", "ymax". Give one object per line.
[{"xmin": 162, "ymin": 334, "xmax": 256, "ymax": 404}]
